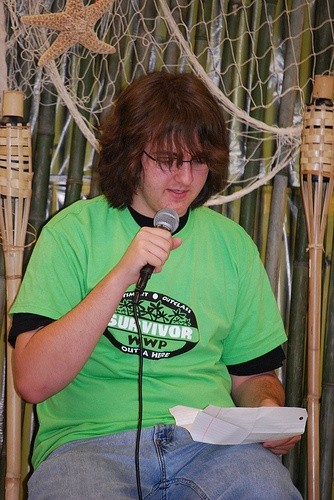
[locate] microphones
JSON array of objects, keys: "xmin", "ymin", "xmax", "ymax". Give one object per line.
[{"xmin": 134, "ymin": 208, "xmax": 179, "ymax": 305}]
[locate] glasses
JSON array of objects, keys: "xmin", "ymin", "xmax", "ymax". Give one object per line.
[{"xmin": 142, "ymin": 149, "xmax": 209, "ymax": 173}]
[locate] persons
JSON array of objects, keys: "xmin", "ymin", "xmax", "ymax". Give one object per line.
[{"xmin": 7, "ymin": 72, "xmax": 304, "ymax": 499}]
